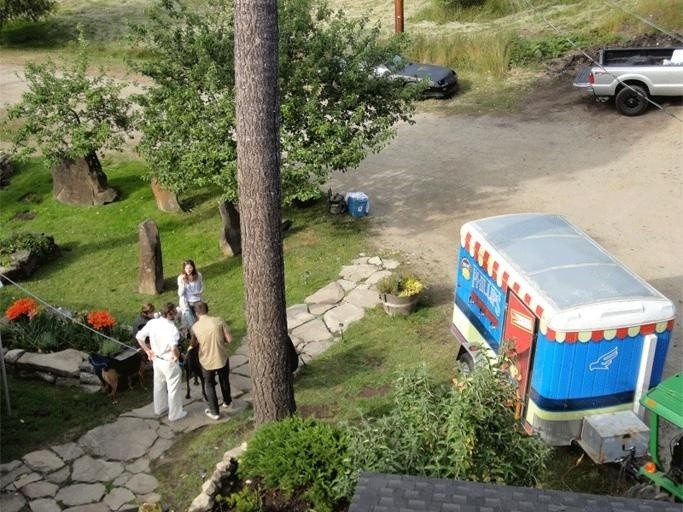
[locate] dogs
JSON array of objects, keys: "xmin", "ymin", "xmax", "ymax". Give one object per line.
[
  {"xmin": 101, "ymin": 348, "xmax": 149, "ymax": 406},
  {"xmin": 185, "ymin": 347, "xmax": 210, "ymax": 403}
]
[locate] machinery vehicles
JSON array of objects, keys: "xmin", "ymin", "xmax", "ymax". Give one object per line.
[{"xmin": 609, "ymin": 369, "xmax": 682, "ymax": 507}]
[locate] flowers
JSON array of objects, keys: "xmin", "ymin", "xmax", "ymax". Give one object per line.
[
  {"xmin": 85, "ymin": 310, "xmax": 115, "ymax": 331},
  {"xmin": 4, "ymin": 296, "xmax": 38, "ymax": 321}
]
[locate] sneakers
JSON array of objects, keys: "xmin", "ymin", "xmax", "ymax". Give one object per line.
[
  {"xmin": 156, "ymin": 409, "xmax": 170, "ymax": 418},
  {"xmin": 204, "ymin": 407, "xmax": 221, "ymax": 420},
  {"xmin": 222, "ymin": 401, "xmax": 234, "ymax": 409},
  {"xmin": 168, "ymin": 410, "xmax": 188, "ymax": 421}
]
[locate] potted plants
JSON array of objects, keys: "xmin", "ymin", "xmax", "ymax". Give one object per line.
[{"xmin": 379, "ymin": 273, "xmax": 422, "ymax": 316}]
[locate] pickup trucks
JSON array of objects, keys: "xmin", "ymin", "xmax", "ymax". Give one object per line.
[{"xmin": 569, "ymin": 42, "xmax": 682, "ymax": 117}]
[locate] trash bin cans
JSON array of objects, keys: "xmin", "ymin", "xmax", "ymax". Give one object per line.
[{"xmin": 347, "ymin": 191, "xmax": 368, "ymax": 219}]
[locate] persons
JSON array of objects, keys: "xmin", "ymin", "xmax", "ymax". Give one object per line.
[
  {"xmin": 189, "ymin": 300, "xmax": 234, "ymax": 420},
  {"xmin": 135, "ymin": 302, "xmax": 189, "ymax": 422},
  {"xmin": 131, "ymin": 302, "xmax": 156, "ymax": 394},
  {"xmin": 288, "ymin": 335, "xmax": 298, "ymax": 372},
  {"xmin": 176, "ymin": 259, "xmax": 204, "ymax": 337}
]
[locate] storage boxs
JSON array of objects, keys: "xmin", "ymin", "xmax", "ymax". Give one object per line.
[{"xmin": 344, "ymin": 192, "xmax": 370, "ymax": 217}]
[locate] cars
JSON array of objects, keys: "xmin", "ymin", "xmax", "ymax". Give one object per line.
[{"xmin": 303, "ymin": 52, "xmax": 458, "ymax": 100}]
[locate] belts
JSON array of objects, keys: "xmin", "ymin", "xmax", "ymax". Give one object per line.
[{"xmin": 156, "ymin": 355, "xmax": 164, "ymax": 360}]
